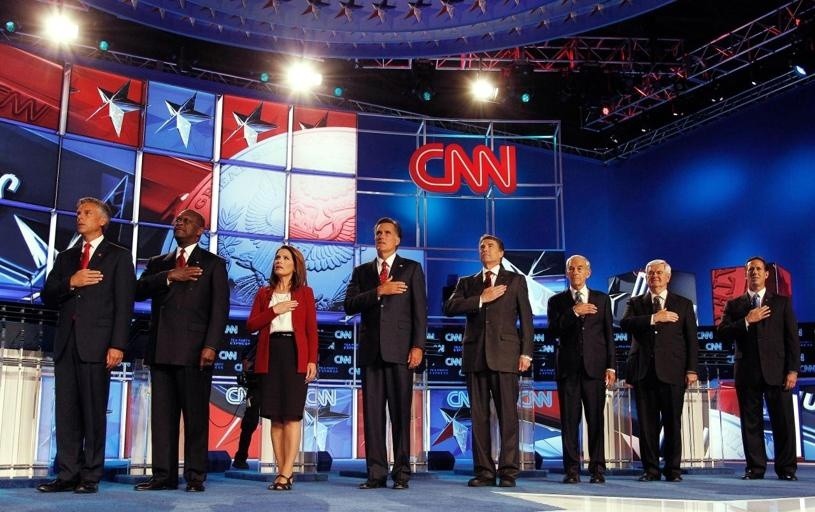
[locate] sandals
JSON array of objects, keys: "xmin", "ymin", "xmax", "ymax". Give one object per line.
[{"xmin": 268, "ymin": 471, "xmax": 295, "ymax": 489}]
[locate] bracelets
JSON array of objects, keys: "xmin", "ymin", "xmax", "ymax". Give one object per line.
[{"xmin": 687, "ymin": 372, "xmax": 698, "ymax": 375}]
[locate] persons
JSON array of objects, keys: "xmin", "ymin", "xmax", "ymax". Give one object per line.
[
  {"xmin": 344, "ymin": 217, "xmax": 426, "ymax": 489},
  {"xmin": 134, "ymin": 209, "xmax": 229, "ymax": 492},
  {"xmin": 444, "ymin": 234, "xmax": 533, "ymax": 487},
  {"xmin": 718, "ymin": 257, "xmax": 801, "ymax": 481},
  {"xmin": 37, "ymin": 196, "xmax": 134, "ymax": 493},
  {"xmin": 246, "ymin": 245, "xmax": 318, "ymax": 490},
  {"xmin": 547, "ymin": 254, "xmax": 616, "ymax": 483},
  {"xmin": 233, "ymin": 333, "xmax": 261, "ymax": 470},
  {"xmin": 619, "ymin": 259, "xmax": 699, "ymax": 482}
]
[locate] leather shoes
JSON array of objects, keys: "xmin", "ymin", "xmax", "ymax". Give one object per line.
[
  {"xmin": 666, "ymin": 472, "xmax": 683, "ymax": 482},
  {"xmin": 564, "ymin": 473, "xmax": 581, "ymax": 485},
  {"xmin": 35, "ymin": 477, "xmax": 80, "ymax": 492},
  {"xmin": 185, "ymin": 481, "xmax": 205, "ymax": 491},
  {"xmin": 74, "ymin": 480, "xmax": 98, "ymax": 493},
  {"xmin": 776, "ymin": 472, "xmax": 798, "ymax": 481},
  {"xmin": 591, "ymin": 472, "xmax": 606, "ymax": 482},
  {"xmin": 359, "ymin": 478, "xmax": 388, "ymax": 489},
  {"xmin": 743, "ymin": 470, "xmax": 765, "ymax": 480},
  {"xmin": 392, "ymin": 480, "xmax": 409, "ymax": 489},
  {"xmin": 639, "ymin": 471, "xmax": 662, "ymax": 481},
  {"xmin": 500, "ymin": 475, "xmax": 515, "ymax": 488},
  {"xmin": 134, "ymin": 474, "xmax": 178, "ymax": 489},
  {"xmin": 468, "ymin": 474, "xmax": 496, "ymax": 487}
]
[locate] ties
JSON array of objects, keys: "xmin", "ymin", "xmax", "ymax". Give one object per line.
[
  {"xmin": 379, "ymin": 261, "xmax": 389, "ymax": 285},
  {"xmin": 176, "ymin": 248, "xmax": 185, "ymax": 269},
  {"xmin": 484, "ymin": 271, "xmax": 492, "ymax": 288},
  {"xmin": 752, "ymin": 294, "xmax": 760, "ymax": 309},
  {"xmin": 653, "ymin": 296, "xmax": 663, "ymax": 312},
  {"xmin": 81, "ymin": 242, "xmax": 91, "ymax": 270},
  {"xmin": 574, "ymin": 291, "xmax": 585, "ymax": 323}
]
